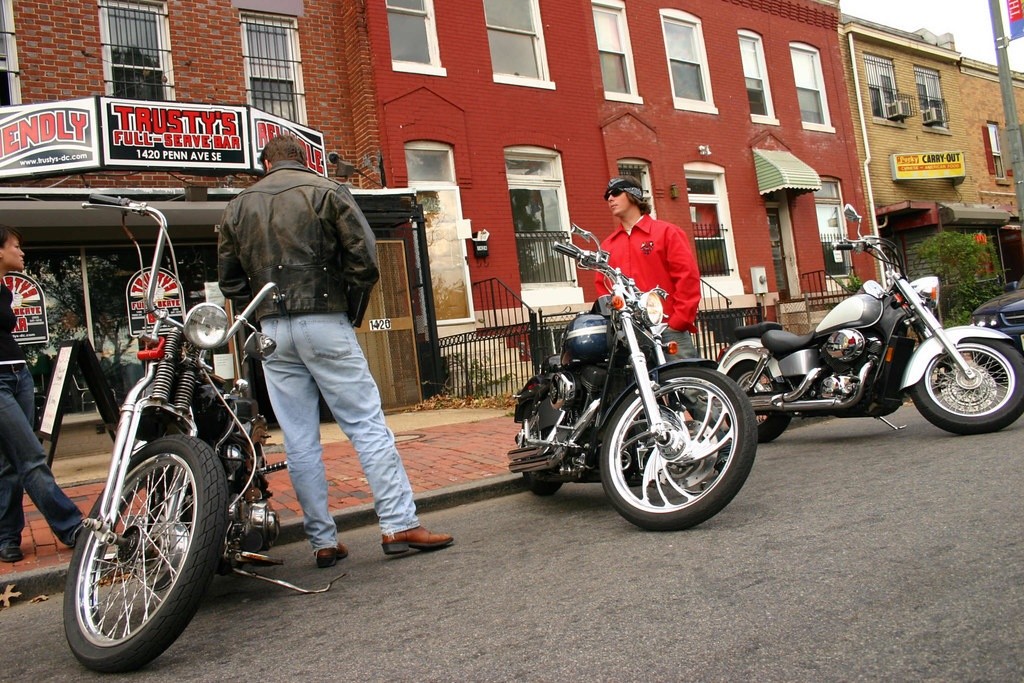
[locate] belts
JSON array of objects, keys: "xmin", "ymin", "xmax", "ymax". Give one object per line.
[{"xmin": 0, "ymin": 363, "xmax": 24, "ymax": 374}]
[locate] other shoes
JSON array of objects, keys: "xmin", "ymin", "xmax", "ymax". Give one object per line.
[
  {"xmin": 628, "ymin": 475, "xmax": 643, "ymax": 486},
  {"xmin": 717, "ymin": 462, "xmax": 725, "ymax": 474}
]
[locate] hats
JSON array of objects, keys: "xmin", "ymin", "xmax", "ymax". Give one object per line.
[{"xmin": 606, "ymin": 176, "xmax": 643, "ymax": 200}]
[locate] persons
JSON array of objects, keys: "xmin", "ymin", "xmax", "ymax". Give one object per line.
[
  {"xmin": 0, "ymin": 225, "xmax": 86, "ymax": 564},
  {"xmin": 593, "ymin": 176, "xmax": 731, "ymax": 475},
  {"xmin": 216, "ymin": 137, "xmax": 453, "ymax": 567}
]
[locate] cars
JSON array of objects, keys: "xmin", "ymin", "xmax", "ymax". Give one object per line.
[{"xmin": 967, "ymin": 289, "xmax": 1024, "ymax": 363}]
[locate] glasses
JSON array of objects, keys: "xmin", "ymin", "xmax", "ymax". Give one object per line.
[{"xmin": 604, "ymin": 188, "xmax": 626, "ymax": 201}]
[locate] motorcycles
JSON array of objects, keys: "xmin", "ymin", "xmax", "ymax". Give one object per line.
[
  {"xmin": 508, "ymin": 221, "xmax": 759, "ymax": 525},
  {"xmin": 61, "ymin": 194, "xmax": 350, "ymax": 677},
  {"xmin": 714, "ymin": 202, "xmax": 1024, "ymax": 438}
]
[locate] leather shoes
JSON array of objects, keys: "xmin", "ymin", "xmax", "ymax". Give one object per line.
[
  {"xmin": 314, "ymin": 544, "xmax": 348, "ymax": 568},
  {"xmin": 0, "ymin": 547, "xmax": 22, "ymax": 561},
  {"xmin": 382, "ymin": 525, "xmax": 453, "ymax": 554}
]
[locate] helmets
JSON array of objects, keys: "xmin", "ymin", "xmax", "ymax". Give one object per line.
[{"xmin": 564, "ymin": 313, "xmax": 609, "ymax": 363}]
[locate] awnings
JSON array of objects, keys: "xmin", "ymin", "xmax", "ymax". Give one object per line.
[{"xmin": 751, "ymin": 148, "xmax": 821, "ymax": 195}]
